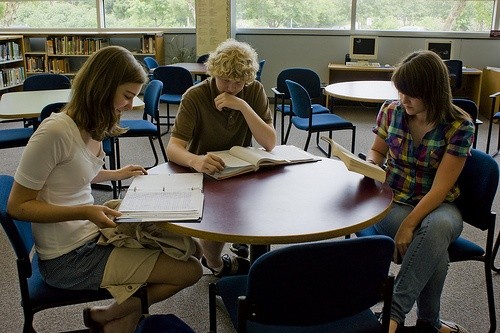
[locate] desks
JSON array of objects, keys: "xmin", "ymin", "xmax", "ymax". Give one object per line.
[
  {"xmin": 479, "ymin": 66, "xmax": 500, "ymax": 123},
  {"xmin": 143, "ymin": 149, "xmax": 393, "ymax": 319},
  {"xmin": 326, "ymin": 62, "xmax": 483, "ymax": 112},
  {"xmin": 150, "ymin": 63, "xmax": 211, "ymax": 82},
  {"xmin": 0, "ymin": 88, "xmax": 146, "ymax": 132},
  {"xmin": 323, "ymin": 79, "xmax": 402, "ymax": 104}
]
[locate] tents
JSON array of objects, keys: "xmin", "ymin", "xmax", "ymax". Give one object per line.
[{"xmin": 0, "ymin": 41, "xmax": 19, "ymax": 62}]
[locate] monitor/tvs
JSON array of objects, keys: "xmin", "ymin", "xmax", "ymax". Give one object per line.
[
  {"xmin": 425, "ymin": 39, "xmax": 454, "ymax": 62},
  {"xmin": 349, "ymin": 35, "xmax": 378, "ymax": 63}
]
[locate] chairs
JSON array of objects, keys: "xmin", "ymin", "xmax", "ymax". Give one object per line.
[{"xmin": 0, "ymin": 53, "xmax": 500, "ymax": 333}]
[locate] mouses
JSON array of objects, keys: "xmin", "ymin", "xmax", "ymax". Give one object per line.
[{"xmin": 385, "ymin": 64, "xmax": 390, "ymax": 68}]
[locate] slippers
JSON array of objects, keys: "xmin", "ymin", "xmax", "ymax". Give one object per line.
[{"xmin": 83, "ymin": 307, "xmax": 104, "ymax": 329}]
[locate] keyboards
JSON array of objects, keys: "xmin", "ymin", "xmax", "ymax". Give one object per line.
[{"xmin": 346, "ymin": 61, "xmax": 381, "ymax": 67}]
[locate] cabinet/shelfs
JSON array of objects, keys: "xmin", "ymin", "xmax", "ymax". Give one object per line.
[{"xmin": 0, "ymin": 31, "xmax": 164, "ymax": 99}]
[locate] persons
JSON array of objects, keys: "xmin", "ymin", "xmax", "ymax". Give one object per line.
[
  {"xmin": 7, "ymin": 46, "xmax": 204, "ymax": 333},
  {"xmin": 369, "ymin": 50, "xmax": 476, "ymax": 333},
  {"xmin": 166, "ymin": 41, "xmax": 277, "ymax": 277}
]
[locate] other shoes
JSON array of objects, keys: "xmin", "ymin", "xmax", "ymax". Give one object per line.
[
  {"xmin": 379, "ymin": 315, "xmax": 399, "ymax": 333},
  {"xmin": 230, "ymin": 242, "xmax": 249, "ymax": 258},
  {"xmin": 203, "ymin": 254, "xmax": 250, "ymax": 278},
  {"xmin": 434, "ymin": 319, "xmax": 469, "ymax": 333}
]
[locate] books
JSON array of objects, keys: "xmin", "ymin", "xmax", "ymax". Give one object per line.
[
  {"xmin": 48, "ymin": 58, "xmax": 64, "ymax": 74},
  {"xmin": 205, "ymin": 145, "xmax": 322, "ymax": 181},
  {"xmin": 27, "ymin": 56, "xmax": 44, "ymax": 72},
  {"xmin": 113, "ymin": 172, "xmax": 205, "ymax": 222},
  {"xmin": 321, "ymin": 136, "xmax": 387, "ymax": 184},
  {"xmin": 0, "ymin": 67, "xmax": 24, "ymax": 88},
  {"xmin": 47, "ymin": 36, "xmax": 107, "ymax": 55}
]
